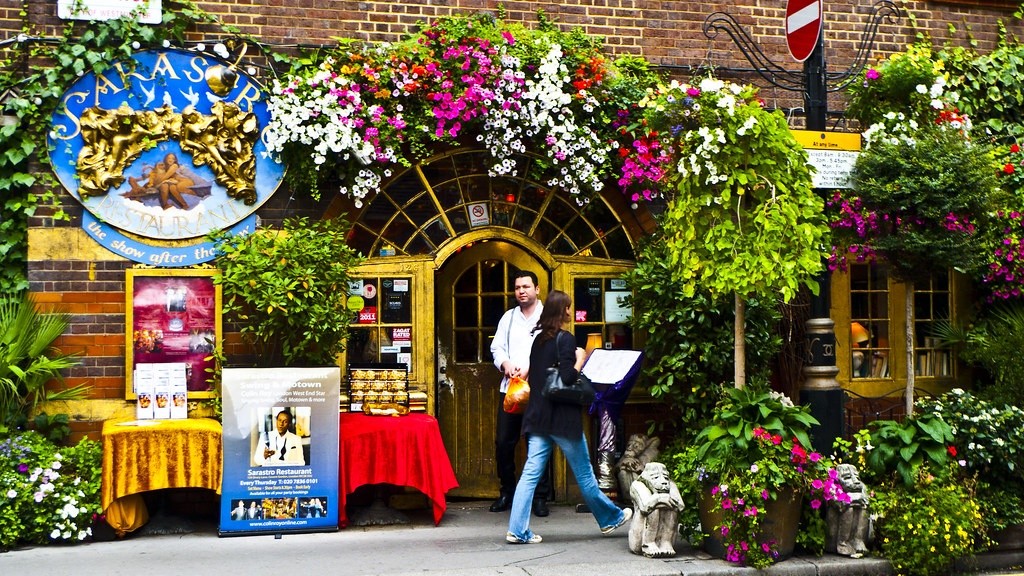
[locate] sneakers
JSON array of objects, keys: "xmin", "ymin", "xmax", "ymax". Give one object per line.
[
  {"xmin": 601, "ymin": 508, "xmax": 632, "ymax": 535},
  {"xmin": 506, "ymin": 531, "xmax": 543, "ymax": 544}
]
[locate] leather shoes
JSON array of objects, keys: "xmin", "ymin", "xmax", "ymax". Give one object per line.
[
  {"xmin": 490, "ymin": 495, "xmax": 512, "ymax": 512},
  {"xmin": 532, "ymin": 498, "xmax": 549, "ymax": 517}
]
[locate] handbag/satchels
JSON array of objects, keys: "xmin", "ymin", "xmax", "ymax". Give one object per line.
[
  {"xmin": 503, "ymin": 372, "xmax": 530, "ymax": 414},
  {"xmin": 542, "ymin": 362, "xmax": 596, "ymax": 407}
]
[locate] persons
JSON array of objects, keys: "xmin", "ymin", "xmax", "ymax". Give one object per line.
[
  {"xmin": 230, "ymin": 499, "xmax": 328, "ymax": 520},
  {"xmin": 252, "ymin": 409, "xmax": 307, "ymax": 468},
  {"xmin": 76, "ymin": 102, "xmax": 260, "ymax": 199},
  {"xmin": 488, "ymin": 270, "xmax": 553, "ymax": 518},
  {"xmin": 504, "ymin": 288, "xmax": 633, "ymax": 545}
]
